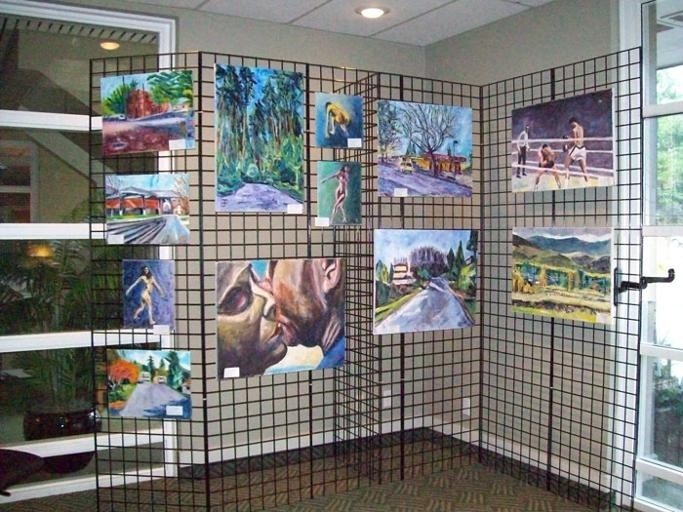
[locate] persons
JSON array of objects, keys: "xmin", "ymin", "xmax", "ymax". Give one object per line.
[
  {"xmin": 320, "ymin": 163, "xmax": 354, "ymax": 224},
  {"xmin": 564, "ymin": 117, "xmax": 590, "ymax": 187},
  {"xmin": 256, "ymin": 258, "xmax": 343, "ymax": 371},
  {"xmin": 532, "ymin": 144, "xmax": 564, "ymax": 191},
  {"xmin": 125, "ymin": 265, "xmax": 164, "ymax": 325},
  {"xmin": 324, "ymin": 101, "xmax": 352, "ymax": 139},
  {"xmin": 217, "ymin": 261, "xmax": 287, "ymax": 379},
  {"xmin": 516, "ymin": 124, "xmax": 529, "ymax": 177}
]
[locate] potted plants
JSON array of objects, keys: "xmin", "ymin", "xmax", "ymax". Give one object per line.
[{"xmin": 0, "ymin": 198, "xmax": 140, "ymax": 475}]
